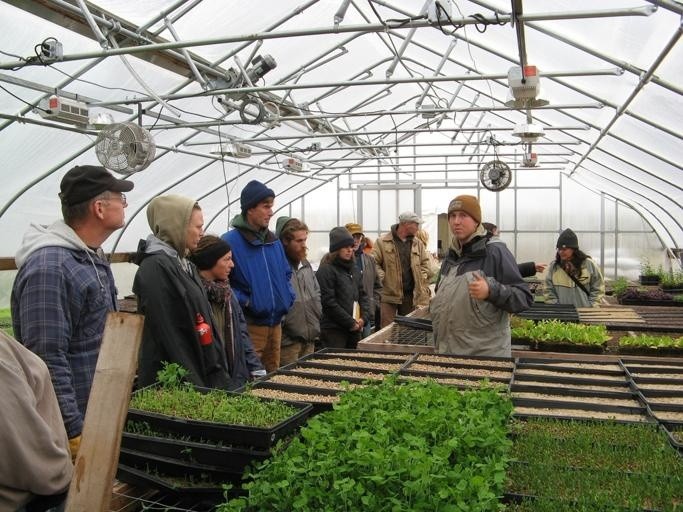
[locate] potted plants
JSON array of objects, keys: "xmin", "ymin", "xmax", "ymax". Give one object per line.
[{"xmin": 114, "ymin": 361, "xmax": 313, "ymax": 500}]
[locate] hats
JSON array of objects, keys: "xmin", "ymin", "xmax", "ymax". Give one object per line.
[
  {"xmin": 345, "ymin": 223, "xmax": 364, "ymax": 236},
  {"xmin": 240, "ymin": 181, "xmax": 275, "ymax": 211},
  {"xmin": 399, "ymin": 211, "xmax": 426, "ymax": 224},
  {"xmin": 329, "ymin": 226, "xmax": 355, "ymax": 253},
  {"xmin": 58, "ymin": 166, "xmax": 135, "ymax": 207},
  {"xmin": 448, "ymin": 195, "xmax": 481, "ymax": 224},
  {"xmin": 557, "ymin": 228, "xmax": 578, "ymax": 249}
]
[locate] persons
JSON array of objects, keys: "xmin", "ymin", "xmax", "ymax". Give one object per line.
[
  {"xmin": 188, "ymin": 234, "xmax": 267, "ymax": 388},
  {"xmin": 0, "ymin": 329, "xmax": 74, "ymax": 512},
  {"xmin": 430, "ymin": 194, "xmax": 535, "ymax": 359},
  {"xmin": 482, "ymin": 222, "xmax": 549, "ymax": 278},
  {"xmin": 10, "ymin": 164, "xmax": 134, "ymax": 459},
  {"xmin": 220, "ymin": 179, "xmax": 297, "ymax": 373},
  {"xmin": 131, "ymin": 194, "xmax": 233, "ymax": 391},
  {"xmin": 542, "ymin": 227, "xmax": 606, "ymax": 309},
  {"xmin": 316, "ymin": 210, "xmax": 443, "ymax": 350},
  {"xmin": 273, "ymin": 215, "xmax": 323, "ymax": 367}
]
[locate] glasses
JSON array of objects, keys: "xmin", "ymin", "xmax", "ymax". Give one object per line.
[{"xmin": 93, "ymin": 194, "xmax": 127, "ymax": 208}]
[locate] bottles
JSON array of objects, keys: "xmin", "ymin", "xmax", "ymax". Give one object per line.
[{"xmin": 194, "ymin": 313, "xmax": 211, "ymax": 346}]
